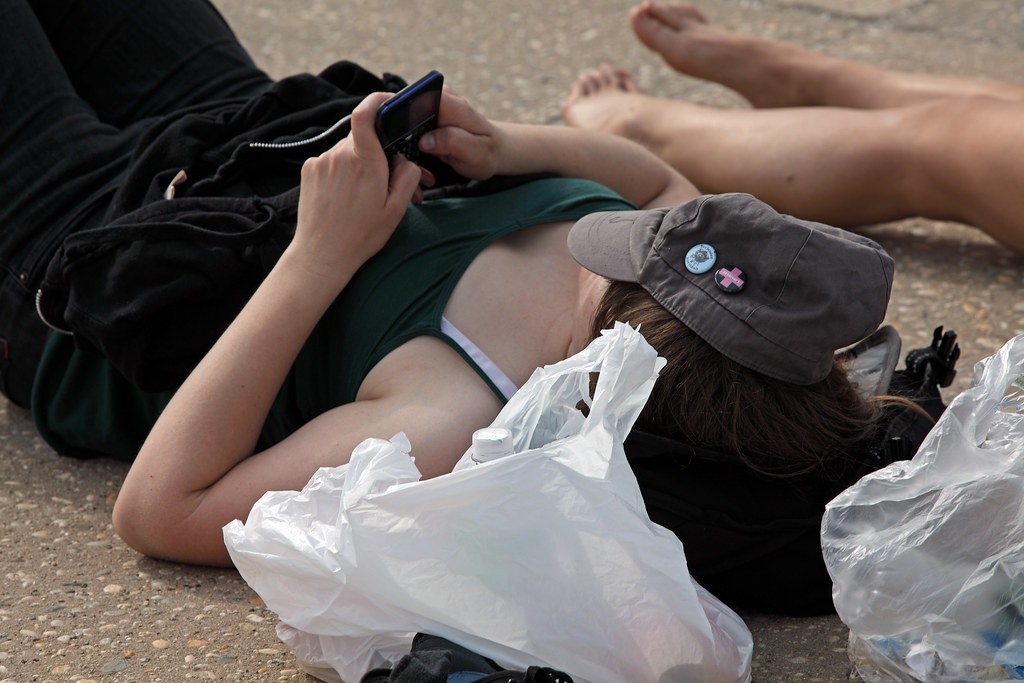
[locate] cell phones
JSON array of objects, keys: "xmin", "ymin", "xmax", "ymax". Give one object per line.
[{"xmin": 375, "ymin": 69, "xmax": 444, "ymax": 175}]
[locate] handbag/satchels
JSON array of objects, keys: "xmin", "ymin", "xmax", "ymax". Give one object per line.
[
  {"xmin": 222, "ymin": 319, "xmax": 754, "ymax": 682},
  {"xmin": 820, "ymin": 331, "xmax": 1024, "ymax": 683}
]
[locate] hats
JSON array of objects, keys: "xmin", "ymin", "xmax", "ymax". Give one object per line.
[{"xmin": 568, "ymin": 191, "xmax": 895, "ymax": 387}]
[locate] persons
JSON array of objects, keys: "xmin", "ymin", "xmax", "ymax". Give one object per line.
[
  {"xmin": 1, "ymin": 0, "xmax": 896, "ymax": 569},
  {"xmin": 564, "ymin": 1, "xmax": 1023, "ymax": 255}
]
